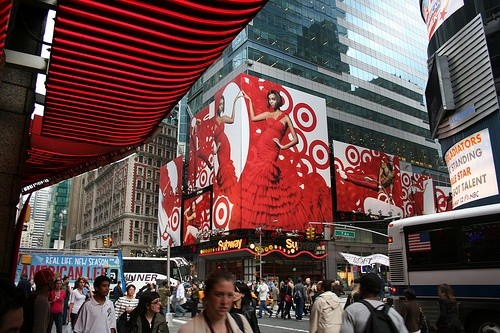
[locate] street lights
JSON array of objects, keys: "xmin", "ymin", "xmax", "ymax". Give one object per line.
[
  {"xmin": 259, "ymin": 219, "xmax": 278, "ymax": 279},
  {"xmin": 56, "ymin": 209, "xmax": 68, "ymax": 254}
]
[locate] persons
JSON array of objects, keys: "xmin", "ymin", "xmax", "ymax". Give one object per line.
[
  {"xmin": 48, "ymin": 275, "xmax": 138, "ymax": 333},
  {"xmin": 338, "ymin": 272, "xmax": 409, "ymax": 333},
  {"xmin": 435, "ymin": 283, "xmax": 462, "ymax": 333},
  {"xmin": 407, "ymin": 175, "xmax": 429, "ymax": 216},
  {"xmin": 227, "ymin": 281, "xmax": 260, "ymax": 333},
  {"xmin": 113, "ymin": 275, "xmax": 359, "ymax": 321},
  {"xmin": 336, "ymin": 155, "xmax": 396, "ymax": 201},
  {"xmin": 0, "ymin": 286, "xmax": 27, "ymax": 333},
  {"xmin": 400, "ymin": 287, "xmax": 422, "ymax": 333},
  {"xmin": 178, "ymin": 271, "xmax": 254, "ymax": 333},
  {"xmin": 239, "ymin": 89, "xmax": 334, "ymax": 234},
  {"xmin": 183, "ymin": 200, "xmax": 203, "ymax": 243},
  {"xmin": 125, "ymin": 291, "xmax": 169, "ymax": 333},
  {"xmin": 189, "ymin": 124, "xmax": 215, "ymax": 190},
  {"xmin": 156, "ymin": 187, "xmax": 179, "ymax": 249},
  {"xmin": 19, "ymin": 267, "xmax": 55, "ymax": 333},
  {"xmin": 213, "ymin": 89, "xmax": 244, "ymax": 197}
]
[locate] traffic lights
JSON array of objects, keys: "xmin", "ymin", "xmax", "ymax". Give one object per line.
[
  {"xmin": 103, "ymin": 237, "xmax": 113, "ymax": 248},
  {"xmin": 306, "ymin": 227, "xmax": 315, "ymax": 240}
]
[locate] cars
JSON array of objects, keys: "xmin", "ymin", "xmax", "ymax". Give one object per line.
[{"xmin": 196, "ymin": 289, "xmax": 275, "ymax": 310}]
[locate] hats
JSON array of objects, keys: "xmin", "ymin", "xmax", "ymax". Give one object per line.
[
  {"xmin": 352, "ymin": 272, "xmax": 383, "ymax": 290},
  {"xmin": 140, "ymin": 292, "xmax": 160, "ymax": 304},
  {"xmin": 33, "ymin": 268, "xmax": 60, "ymax": 285}
]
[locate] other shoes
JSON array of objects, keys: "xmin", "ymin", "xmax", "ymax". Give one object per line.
[{"xmin": 252, "ymin": 307, "xmax": 308, "ymax": 323}]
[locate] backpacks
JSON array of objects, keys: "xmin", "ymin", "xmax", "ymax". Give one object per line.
[
  {"xmin": 358, "ymin": 301, "xmax": 400, "ymax": 333},
  {"xmin": 448, "ymin": 300, "xmax": 463, "ymax": 328}
]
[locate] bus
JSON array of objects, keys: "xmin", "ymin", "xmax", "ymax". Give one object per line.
[
  {"xmin": 386, "ymin": 202, "xmax": 500, "ymax": 333},
  {"xmin": 106, "ymin": 257, "xmax": 194, "ymax": 299}
]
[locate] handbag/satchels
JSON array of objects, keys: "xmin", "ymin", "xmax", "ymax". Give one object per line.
[
  {"xmin": 116, "ymin": 310, "xmax": 130, "ymax": 333},
  {"xmin": 417, "ymin": 305, "xmax": 436, "ymax": 333}
]
